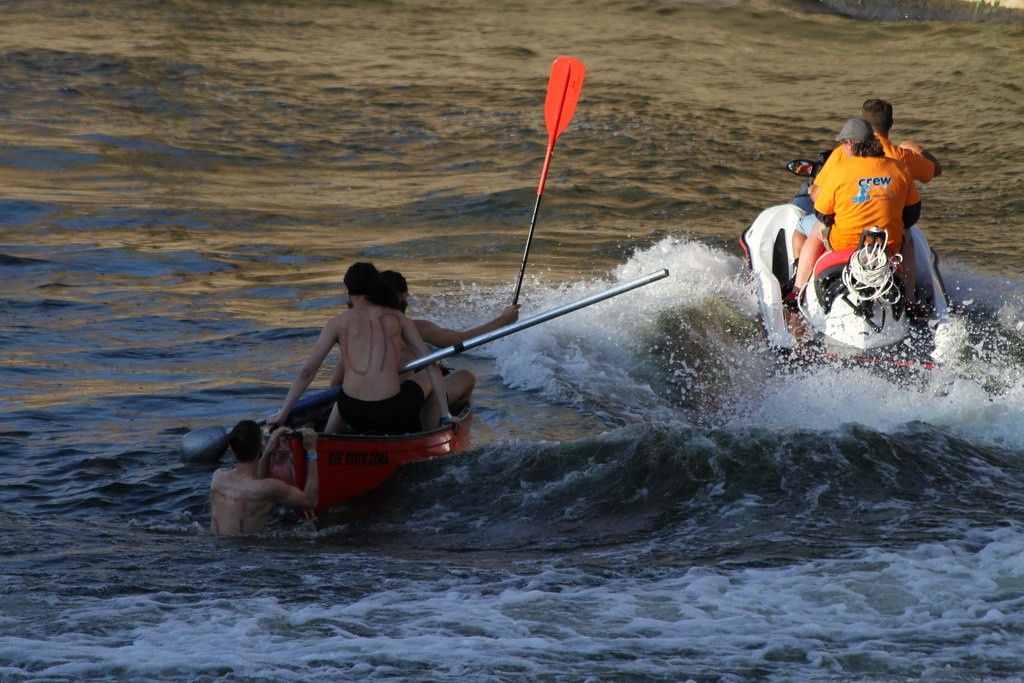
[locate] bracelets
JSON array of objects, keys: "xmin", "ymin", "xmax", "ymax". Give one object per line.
[
  {"xmin": 307, "ymin": 455, "xmax": 317, "ymax": 459},
  {"xmin": 306, "ymin": 449, "xmax": 318, "ymax": 454}
]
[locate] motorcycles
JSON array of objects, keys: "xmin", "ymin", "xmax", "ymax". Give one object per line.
[{"xmin": 738, "ymin": 148, "xmax": 957, "ymax": 385}]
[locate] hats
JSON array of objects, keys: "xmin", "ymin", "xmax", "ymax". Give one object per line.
[
  {"xmin": 834, "ymin": 118, "xmax": 874, "ymax": 142},
  {"xmin": 344, "ymin": 262, "xmax": 379, "ymax": 288},
  {"xmin": 380, "ymin": 270, "xmax": 408, "ymax": 293}
]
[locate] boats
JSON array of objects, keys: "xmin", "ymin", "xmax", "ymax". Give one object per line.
[{"xmin": 260, "ymin": 344, "xmax": 474, "ymax": 521}]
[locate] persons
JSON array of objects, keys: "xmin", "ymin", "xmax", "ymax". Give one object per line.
[
  {"xmin": 208, "ymin": 261, "xmax": 521, "ymax": 537},
  {"xmin": 786, "ymin": 98, "xmax": 942, "ymax": 338}
]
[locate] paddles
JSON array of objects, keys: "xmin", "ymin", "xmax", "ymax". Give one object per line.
[{"xmin": 513, "ymin": 55, "xmax": 586, "ymax": 303}]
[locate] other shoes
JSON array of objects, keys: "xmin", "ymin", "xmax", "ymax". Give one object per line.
[
  {"xmin": 906, "ymin": 306, "xmax": 930, "ymax": 325},
  {"xmin": 782, "ymin": 298, "xmax": 802, "ymax": 313}
]
